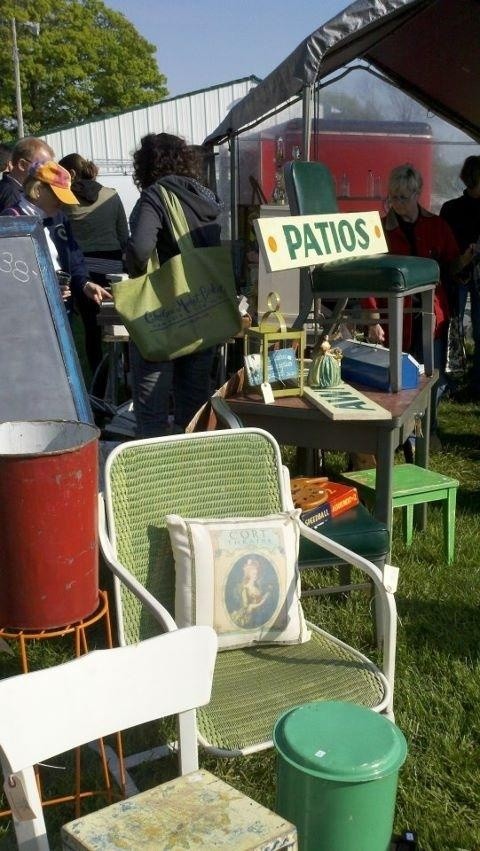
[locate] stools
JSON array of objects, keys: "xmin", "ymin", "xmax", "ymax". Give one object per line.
[
  {"xmin": 339, "ymin": 462, "xmax": 458, "ymax": 566},
  {"xmin": 1, "ymin": 590, "xmax": 125, "ymax": 827},
  {"xmin": 62, "ymin": 770, "xmax": 297, "ymax": 851}
]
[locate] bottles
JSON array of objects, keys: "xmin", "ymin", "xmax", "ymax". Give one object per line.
[
  {"xmin": 342, "ymin": 171, "xmax": 350, "ymax": 197},
  {"xmin": 366, "ymin": 168, "xmax": 374, "ymax": 199}
]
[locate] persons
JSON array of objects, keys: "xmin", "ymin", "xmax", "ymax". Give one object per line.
[
  {"xmin": 437, "ymin": 155, "xmax": 480, "ymax": 369},
  {"xmin": 59, "ymin": 153, "xmax": 129, "ymax": 400},
  {"xmin": 1, "ymin": 138, "xmax": 54, "ymax": 212},
  {"xmin": 0, "ymin": 160, "xmax": 113, "ymax": 394},
  {"xmin": 229, "ymin": 562, "xmax": 269, "ymax": 627},
  {"xmin": 125, "ymin": 133, "xmax": 225, "ymax": 440},
  {"xmin": 362, "ymin": 164, "xmax": 458, "ymax": 392}
]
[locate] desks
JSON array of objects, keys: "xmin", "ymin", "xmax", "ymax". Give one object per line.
[{"xmin": 226, "ymin": 367, "xmax": 440, "ymax": 569}]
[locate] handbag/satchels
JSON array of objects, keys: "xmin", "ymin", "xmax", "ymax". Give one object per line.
[
  {"xmin": 109, "ymin": 185, "xmax": 241, "ymax": 361},
  {"xmin": 443, "ymin": 264, "xmax": 468, "ymax": 378}
]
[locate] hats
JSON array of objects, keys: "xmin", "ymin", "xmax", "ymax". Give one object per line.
[{"xmin": 30, "ymin": 160, "xmax": 81, "ymax": 206}]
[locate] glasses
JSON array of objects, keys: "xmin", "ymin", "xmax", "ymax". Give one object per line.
[{"xmin": 389, "ymin": 190, "xmax": 417, "ymax": 203}]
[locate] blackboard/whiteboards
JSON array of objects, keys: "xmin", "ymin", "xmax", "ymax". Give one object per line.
[{"xmin": 0, "ymin": 214, "xmax": 97, "ymax": 427}]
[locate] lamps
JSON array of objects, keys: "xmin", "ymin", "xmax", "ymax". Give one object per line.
[{"xmin": 244, "ymin": 292, "xmax": 304, "ymax": 398}]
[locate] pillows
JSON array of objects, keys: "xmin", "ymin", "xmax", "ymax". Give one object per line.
[{"xmin": 164, "ymin": 507, "xmax": 313, "ymax": 653}]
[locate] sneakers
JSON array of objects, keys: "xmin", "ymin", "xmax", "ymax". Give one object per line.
[{"xmin": 430, "ymin": 432, "xmax": 442, "ymax": 453}]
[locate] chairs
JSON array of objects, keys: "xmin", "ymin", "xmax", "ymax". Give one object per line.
[
  {"xmin": 96, "ymin": 427, "xmax": 397, "ymax": 794},
  {"xmin": 210, "ymin": 396, "xmax": 390, "ymax": 597},
  {"xmin": 281, "ymin": 161, "xmax": 440, "ymax": 395}
]
